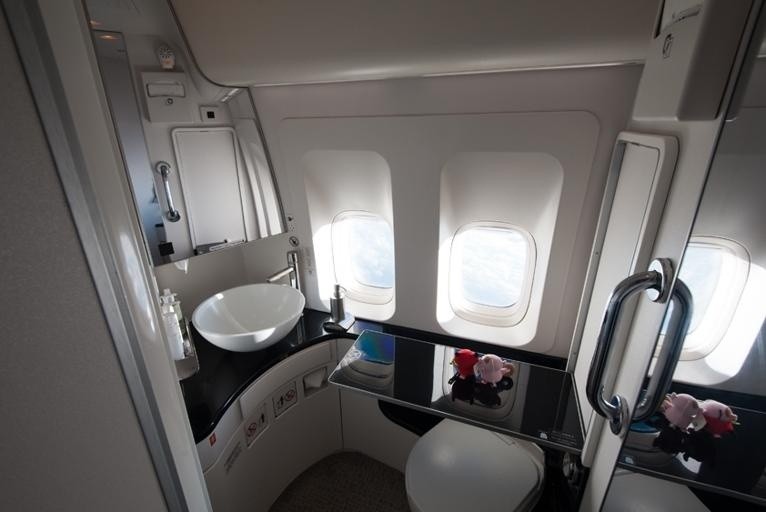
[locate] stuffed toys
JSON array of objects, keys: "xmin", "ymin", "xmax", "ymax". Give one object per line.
[
  {"xmin": 473, "ymin": 353, "xmax": 515, "ymax": 391},
  {"xmin": 700, "ymin": 398, "xmax": 738, "ymax": 439},
  {"xmin": 661, "ymin": 391, "xmax": 710, "ymax": 442},
  {"xmin": 453, "ymin": 347, "xmax": 480, "ymax": 381}
]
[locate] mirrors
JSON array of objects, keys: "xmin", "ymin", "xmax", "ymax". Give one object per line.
[{"xmin": 85, "ymin": 0, "xmax": 289, "ymax": 267}]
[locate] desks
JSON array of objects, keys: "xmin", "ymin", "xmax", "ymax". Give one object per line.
[
  {"xmin": 615, "ymin": 379, "xmax": 766, "ymax": 499},
  {"xmin": 328, "ymin": 327, "xmax": 585, "ymax": 459}
]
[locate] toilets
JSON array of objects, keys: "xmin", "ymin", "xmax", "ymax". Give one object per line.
[{"xmin": 404, "ymin": 417, "xmax": 548, "ymax": 511}]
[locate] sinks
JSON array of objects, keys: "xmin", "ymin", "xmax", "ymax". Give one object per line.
[{"xmin": 192, "ymin": 283, "xmax": 306, "ymax": 352}]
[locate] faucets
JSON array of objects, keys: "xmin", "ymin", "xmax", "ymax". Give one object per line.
[{"xmin": 267, "ymin": 250, "xmax": 300, "ymax": 289}]
